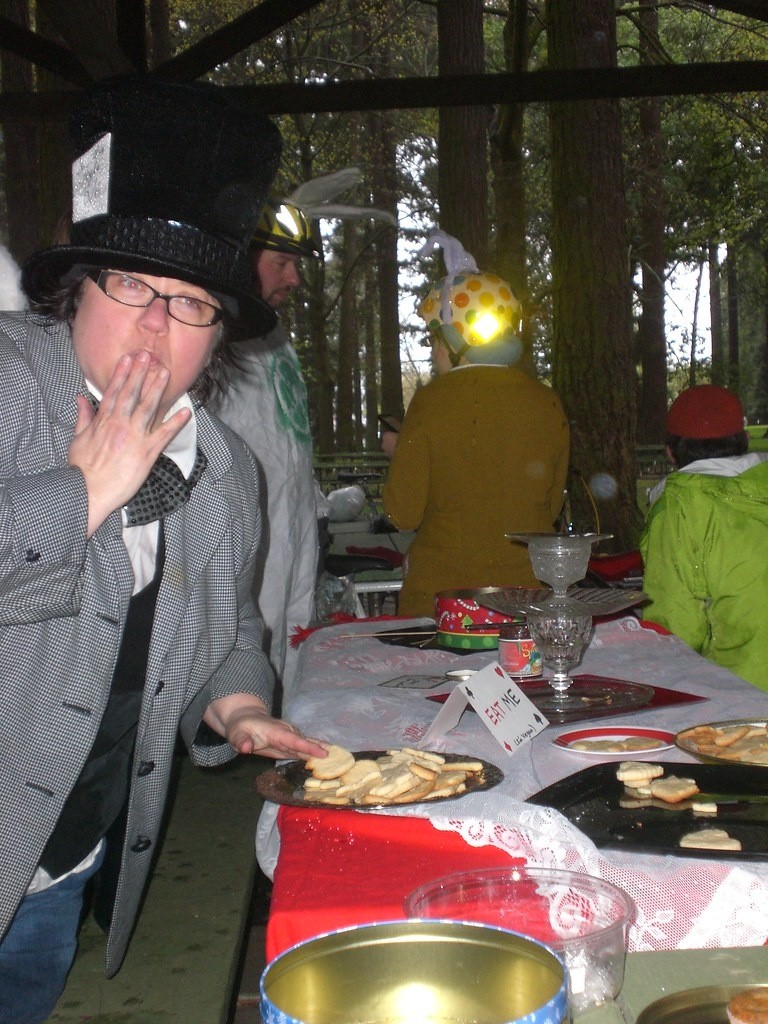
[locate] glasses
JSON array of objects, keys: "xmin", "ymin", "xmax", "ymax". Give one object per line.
[{"xmin": 87, "ymin": 264, "xmax": 223, "ymax": 328}]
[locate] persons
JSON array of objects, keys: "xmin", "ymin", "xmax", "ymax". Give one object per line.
[
  {"xmin": 0, "ymin": 79, "xmax": 329, "ymax": 1024},
  {"xmin": 640, "ymin": 384, "xmax": 768, "ymax": 688},
  {"xmin": 206, "ymin": 196, "xmax": 317, "ymax": 701},
  {"xmin": 382, "ymin": 266, "xmax": 572, "ymax": 615}
]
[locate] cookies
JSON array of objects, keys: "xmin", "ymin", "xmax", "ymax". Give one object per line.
[
  {"xmin": 573, "ymin": 723, "xmax": 768, "ymax": 850},
  {"xmin": 304, "ymin": 746, "xmax": 484, "ymax": 804}
]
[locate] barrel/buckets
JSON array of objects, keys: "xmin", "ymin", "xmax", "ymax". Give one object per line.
[{"xmin": 257, "ymin": 921, "xmax": 572, "ymax": 1024}]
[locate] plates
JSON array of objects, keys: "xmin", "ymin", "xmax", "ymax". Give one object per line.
[
  {"xmin": 252, "ymin": 751, "xmax": 504, "ymax": 807},
  {"xmin": 675, "ymin": 719, "xmax": 768, "ymax": 767},
  {"xmin": 637, "ymin": 983, "xmax": 768, "ymax": 1024},
  {"xmin": 520, "ymin": 762, "xmax": 768, "ymax": 862},
  {"xmin": 476, "ymin": 589, "xmax": 648, "ymax": 618},
  {"xmin": 550, "ymin": 727, "xmax": 676, "ymax": 759}
]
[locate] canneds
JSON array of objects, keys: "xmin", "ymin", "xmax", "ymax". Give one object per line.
[{"xmin": 499, "ymin": 626, "xmax": 544, "ymax": 681}]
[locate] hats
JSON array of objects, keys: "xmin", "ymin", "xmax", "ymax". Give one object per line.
[
  {"xmin": 666, "ymin": 385, "xmax": 744, "ymax": 439},
  {"xmin": 19, "ymin": 67, "xmax": 278, "ymax": 339}
]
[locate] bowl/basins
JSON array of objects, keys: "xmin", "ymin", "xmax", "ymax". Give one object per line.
[{"xmin": 402, "ymin": 868, "xmax": 636, "ymax": 1014}]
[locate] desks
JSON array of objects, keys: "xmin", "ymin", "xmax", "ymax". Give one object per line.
[
  {"xmin": 225, "ymin": 614, "xmax": 768, "ymax": 1024},
  {"xmin": 314, "ymin": 460, "xmax": 389, "ymax": 507},
  {"xmin": 317, "ymin": 451, "xmax": 390, "ymax": 496}
]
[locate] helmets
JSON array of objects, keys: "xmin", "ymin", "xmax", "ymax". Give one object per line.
[
  {"xmin": 416, "ymin": 269, "xmax": 526, "ymax": 365},
  {"xmin": 248, "ymin": 195, "xmax": 324, "ymax": 259}
]
